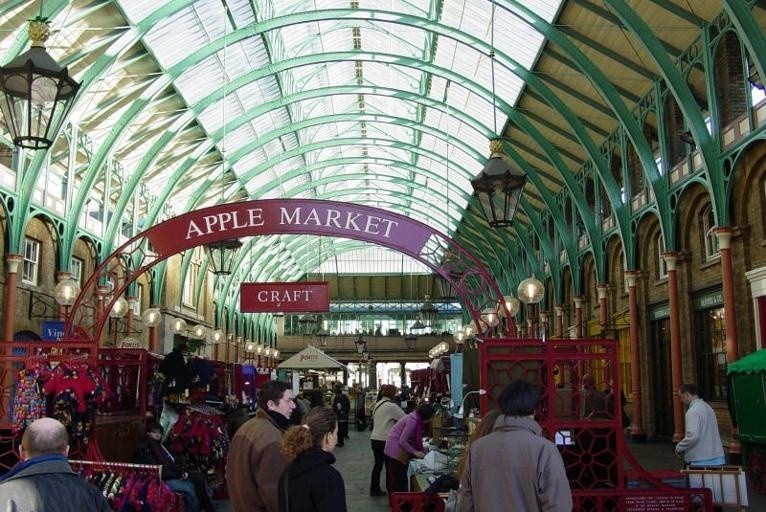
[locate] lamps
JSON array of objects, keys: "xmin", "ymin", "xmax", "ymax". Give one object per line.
[
  {"xmin": 28, "ymin": 280, "xmax": 281, "ymax": 359},
  {"xmin": 470, "ymin": 0, "xmax": 528, "ymax": 228},
  {"xmin": 429, "ymin": 89, "xmax": 462, "ymax": 299},
  {"xmin": 414, "ymin": 294, "xmax": 438, "ymax": 327},
  {"xmin": 203, "ymin": 8, "xmax": 244, "ymax": 275},
  {"xmin": 404, "ymin": 333, "xmax": 418, "ymax": 349},
  {"xmin": 298, "ymin": 312, "xmax": 317, "ymax": 335},
  {"xmin": 315, "ymin": 328, "xmax": 329, "ymax": 346},
  {"xmin": 0, "ymin": 0, "xmax": 84, "ymax": 149},
  {"xmin": 428, "ymin": 278, "xmax": 545, "ymax": 359}
]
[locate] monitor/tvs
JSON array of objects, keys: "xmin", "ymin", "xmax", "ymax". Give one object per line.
[{"xmin": 554, "ymin": 428, "xmax": 575, "ymax": 447}]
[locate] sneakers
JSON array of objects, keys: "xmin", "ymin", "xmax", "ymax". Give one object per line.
[{"xmin": 371, "ymin": 488, "xmax": 386, "ymax": 496}]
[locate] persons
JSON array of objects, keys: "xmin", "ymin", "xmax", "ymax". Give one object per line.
[
  {"xmin": 454, "ymin": 379, "xmax": 575, "ymax": 511},
  {"xmin": 275, "ymin": 403, "xmax": 349, "ymax": 510},
  {"xmin": 368, "ymin": 384, "xmax": 408, "ymax": 496},
  {"xmin": 132, "ymin": 420, "xmax": 214, "ymax": 511},
  {"xmin": 223, "ymin": 380, "xmax": 304, "ymax": 511},
  {"xmin": 0, "ymin": 414, "xmax": 116, "ymax": 511},
  {"xmin": 328, "ymin": 384, "xmax": 350, "ymax": 448},
  {"xmin": 455, "ymin": 407, "xmax": 504, "ymax": 483},
  {"xmin": 376, "ymin": 383, "xmax": 385, "ymax": 403},
  {"xmin": 556, "ymin": 373, "xmax": 633, "ymax": 486},
  {"xmin": 382, "ymin": 403, "xmax": 437, "ymax": 506},
  {"xmin": 672, "ymin": 383, "xmax": 728, "ymax": 468},
  {"xmin": 330, "ymin": 381, "xmax": 350, "ymax": 439},
  {"xmin": 354, "ymin": 385, "xmax": 368, "ymax": 432}
]
